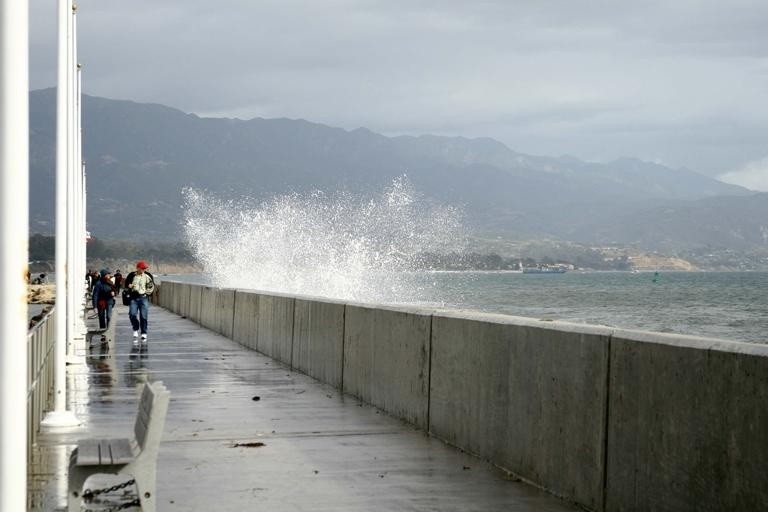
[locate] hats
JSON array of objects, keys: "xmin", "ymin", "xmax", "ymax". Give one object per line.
[
  {"xmin": 100, "ymin": 270, "xmax": 110, "ymax": 276},
  {"xmin": 135, "ymin": 262, "xmax": 148, "ymax": 269}
]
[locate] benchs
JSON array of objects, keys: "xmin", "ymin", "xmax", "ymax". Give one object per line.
[
  {"xmin": 84, "ymin": 300, "xmax": 98, "ymax": 319},
  {"xmin": 87, "ymin": 307, "xmax": 117, "ymax": 357},
  {"xmin": 68, "ymin": 380, "xmax": 170, "ymax": 512}
]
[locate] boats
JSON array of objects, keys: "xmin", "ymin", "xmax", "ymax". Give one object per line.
[{"xmin": 522, "ymin": 263, "xmax": 567, "ymax": 274}]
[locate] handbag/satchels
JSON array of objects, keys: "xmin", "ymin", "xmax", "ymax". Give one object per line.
[{"xmin": 122, "ymin": 289, "xmax": 132, "ymax": 306}]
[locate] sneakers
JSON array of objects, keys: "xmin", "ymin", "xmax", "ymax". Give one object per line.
[
  {"xmin": 133, "ymin": 330, "xmax": 139, "ymax": 338},
  {"xmin": 140, "ymin": 333, "xmax": 146, "ymax": 339}
]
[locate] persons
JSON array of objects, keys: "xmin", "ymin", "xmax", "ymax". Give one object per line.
[
  {"xmin": 88, "ymin": 337, "xmax": 114, "ymax": 402},
  {"xmin": 26, "ymin": 305, "xmax": 50, "ymax": 327},
  {"xmin": 32, "ymin": 272, "xmax": 47, "ymax": 286},
  {"xmin": 86, "ymin": 261, "xmax": 157, "ymax": 340},
  {"xmin": 120, "ymin": 341, "xmax": 155, "ymax": 392}
]
